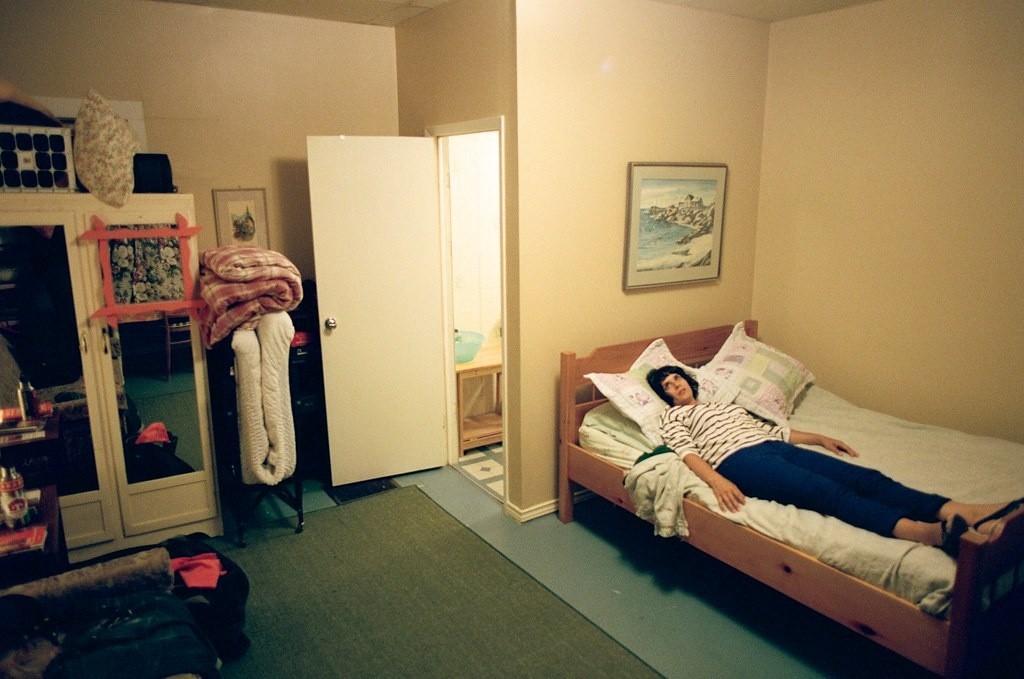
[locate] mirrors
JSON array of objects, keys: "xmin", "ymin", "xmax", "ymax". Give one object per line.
[{"xmin": 0, "ymin": 224, "xmax": 206, "ymax": 498}]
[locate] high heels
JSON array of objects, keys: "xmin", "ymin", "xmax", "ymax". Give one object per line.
[
  {"xmin": 973, "ymin": 495, "xmax": 1024, "ymax": 535},
  {"xmin": 933, "ymin": 513, "xmax": 969, "ymax": 561}
]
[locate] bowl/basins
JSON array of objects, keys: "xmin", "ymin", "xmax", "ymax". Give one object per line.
[{"xmin": 455, "ymin": 331, "xmax": 484, "ymax": 363}]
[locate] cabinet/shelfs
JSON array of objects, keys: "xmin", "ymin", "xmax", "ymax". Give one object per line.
[
  {"xmin": 455, "ymin": 360, "xmax": 503, "ymax": 457},
  {"xmin": 0, "ymin": 193, "xmax": 222, "ymax": 564},
  {"xmin": 210, "ymin": 297, "xmax": 328, "ymax": 547},
  {"xmin": 0, "ymin": 486, "xmax": 71, "ymax": 588}
]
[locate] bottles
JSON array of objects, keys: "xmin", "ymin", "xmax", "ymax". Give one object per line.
[
  {"xmin": 17, "ymin": 372, "xmax": 40, "ymax": 426},
  {"xmin": 0, "ymin": 455, "xmax": 32, "ymax": 528},
  {"xmin": 455, "ymin": 329, "xmax": 463, "ymax": 343}
]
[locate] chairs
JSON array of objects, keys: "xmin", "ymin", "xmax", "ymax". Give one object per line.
[{"xmin": 162, "ymin": 311, "xmax": 191, "ymax": 378}]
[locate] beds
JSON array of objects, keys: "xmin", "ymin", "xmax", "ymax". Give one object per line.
[{"xmin": 558, "ymin": 319, "xmax": 1024, "ymax": 679}]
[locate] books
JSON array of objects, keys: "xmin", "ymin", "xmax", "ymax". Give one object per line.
[
  {"xmin": 0, "ymin": 401, "xmax": 53, "ymax": 447},
  {"xmin": 0, "ymin": 490, "xmax": 47, "ymax": 556}
]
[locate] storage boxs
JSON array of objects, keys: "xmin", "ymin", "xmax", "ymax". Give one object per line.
[{"xmin": 0, "ymin": 125, "xmax": 78, "ymax": 193}]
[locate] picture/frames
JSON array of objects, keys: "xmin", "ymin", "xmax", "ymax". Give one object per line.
[
  {"xmin": 212, "ymin": 189, "xmax": 270, "ymax": 251},
  {"xmin": 622, "ymin": 161, "xmax": 727, "ymax": 291}
]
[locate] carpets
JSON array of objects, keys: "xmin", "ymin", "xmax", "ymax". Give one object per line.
[{"xmin": 207, "ymin": 484, "xmax": 670, "ymax": 679}]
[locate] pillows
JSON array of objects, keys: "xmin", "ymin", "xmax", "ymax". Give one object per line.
[
  {"xmin": 583, "ymin": 337, "xmax": 741, "ymax": 446},
  {"xmin": 700, "ymin": 321, "xmax": 816, "ymax": 426},
  {"xmin": 73, "ymin": 90, "xmax": 140, "ymax": 209}
]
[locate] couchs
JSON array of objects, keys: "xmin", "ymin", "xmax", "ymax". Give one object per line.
[{"xmin": 0, "ymin": 546, "xmax": 212, "ymax": 679}]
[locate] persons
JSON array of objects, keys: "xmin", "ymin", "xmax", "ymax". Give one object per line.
[{"xmin": 646, "ymin": 365, "xmax": 1024, "ymax": 559}]
